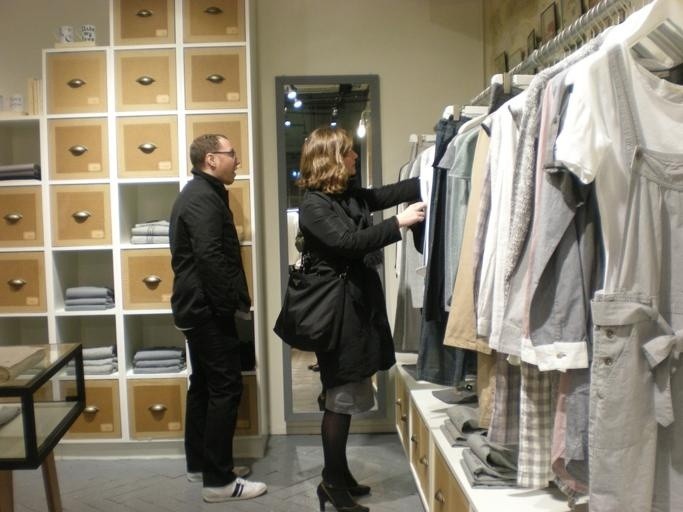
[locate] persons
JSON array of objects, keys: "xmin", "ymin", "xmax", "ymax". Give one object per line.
[
  {"xmin": 289, "ymin": 126, "xmax": 427, "ymax": 512},
  {"xmin": 168, "ymin": 135, "xmax": 267, "ymax": 502}
]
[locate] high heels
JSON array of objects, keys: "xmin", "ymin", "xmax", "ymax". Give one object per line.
[{"xmin": 317, "ymin": 466, "xmax": 370, "ymax": 512}]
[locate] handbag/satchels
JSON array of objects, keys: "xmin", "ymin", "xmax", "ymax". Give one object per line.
[{"xmin": 273, "ymin": 260, "xmax": 362, "ymax": 353}]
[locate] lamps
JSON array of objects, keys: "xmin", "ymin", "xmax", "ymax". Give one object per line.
[{"xmin": 285, "ymin": 84, "xmax": 297, "ymax": 101}]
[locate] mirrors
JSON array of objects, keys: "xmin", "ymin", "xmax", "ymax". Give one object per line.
[{"xmin": 273, "ymin": 74, "xmax": 389, "ymax": 426}]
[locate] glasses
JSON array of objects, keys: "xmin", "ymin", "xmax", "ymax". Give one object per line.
[{"xmin": 213, "ymin": 149, "xmax": 234, "ymax": 156}]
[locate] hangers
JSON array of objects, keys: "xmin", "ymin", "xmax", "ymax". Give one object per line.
[{"xmin": 487, "ymin": 1, "xmax": 681, "ymax": 122}]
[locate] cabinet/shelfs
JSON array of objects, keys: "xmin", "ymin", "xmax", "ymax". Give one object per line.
[
  {"xmin": 391, "ymin": 362, "xmax": 578, "ymax": 512},
  {"xmin": 0, "ymin": 2, "xmax": 269, "ymax": 460}
]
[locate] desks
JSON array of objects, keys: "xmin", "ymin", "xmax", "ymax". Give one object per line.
[{"xmin": 0, "ymin": 342, "xmax": 86, "ymax": 512}]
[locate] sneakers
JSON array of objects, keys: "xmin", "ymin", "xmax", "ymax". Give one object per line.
[{"xmin": 185, "ymin": 466, "xmax": 267, "ymax": 503}]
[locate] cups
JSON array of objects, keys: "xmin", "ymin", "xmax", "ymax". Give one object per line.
[
  {"xmin": 9, "ymin": 95, "xmax": 23, "ymax": 113},
  {"xmin": 52, "ymin": 24, "xmax": 95, "ymax": 43}
]
